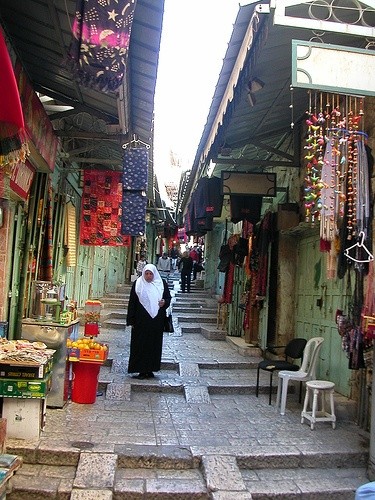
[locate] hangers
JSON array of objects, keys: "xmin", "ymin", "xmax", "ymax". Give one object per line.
[
  {"xmin": 193, "ymin": 169, "xmax": 222, "ymax": 191},
  {"xmin": 343, "ymin": 231, "xmax": 374, "ymax": 262},
  {"xmin": 123, "ymin": 134, "xmax": 152, "ymax": 149}
]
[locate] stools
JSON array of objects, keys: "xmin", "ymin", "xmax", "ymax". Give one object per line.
[{"xmin": 301, "ymin": 381, "xmax": 338, "ymax": 430}]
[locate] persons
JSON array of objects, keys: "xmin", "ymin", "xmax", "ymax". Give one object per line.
[
  {"xmin": 178, "ymin": 251, "xmax": 193, "ymax": 293},
  {"xmin": 169, "ymin": 245, "xmax": 204, "ymax": 281},
  {"xmin": 157, "ymin": 253, "xmax": 171, "ymax": 272},
  {"xmin": 136, "ymin": 254, "xmax": 148, "ymax": 277},
  {"xmin": 126, "ymin": 263, "xmax": 171, "ymax": 380}
]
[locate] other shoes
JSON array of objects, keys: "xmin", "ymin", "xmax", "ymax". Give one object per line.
[{"xmin": 138, "ymin": 372, "xmax": 155, "ymax": 379}]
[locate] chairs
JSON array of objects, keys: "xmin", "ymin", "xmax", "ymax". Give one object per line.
[{"xmin": 256, "ymin": 336, "xmax": 324, "ymax": 416}]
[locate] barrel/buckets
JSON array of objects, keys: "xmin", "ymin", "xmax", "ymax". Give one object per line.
[{"xmin": 71, "ymin": 362, "xmax": 101, "ymax": 403}]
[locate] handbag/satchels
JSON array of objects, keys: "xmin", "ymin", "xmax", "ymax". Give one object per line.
[{"xmin": 163, "ymin": 301, "xmax": 174, "ymax": 332}]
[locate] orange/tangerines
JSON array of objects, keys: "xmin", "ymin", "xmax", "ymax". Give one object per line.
[{"xmin": 66, "ymin": 338, "xmax": 107, "ymax": 351}]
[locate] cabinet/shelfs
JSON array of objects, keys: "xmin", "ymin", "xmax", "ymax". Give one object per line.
[{"xmin": 21, "ymin": 316, "xmax": 81, "ymax": 409}]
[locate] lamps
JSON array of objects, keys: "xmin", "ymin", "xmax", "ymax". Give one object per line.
[{"xmin": 246, "ymin": 79, "xmax": 265, "ymax": 106}]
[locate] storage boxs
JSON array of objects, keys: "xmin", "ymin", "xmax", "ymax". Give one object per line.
[
  {"xmin": 0, "ymin": 351, "xmax": 58, "ymax": 439},
  {"xmin": 80, "ymin": 349, "xmax": 109, "ymax": 359}
]
